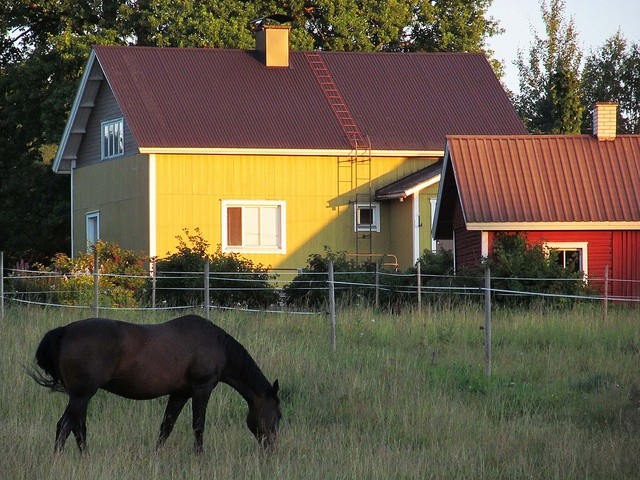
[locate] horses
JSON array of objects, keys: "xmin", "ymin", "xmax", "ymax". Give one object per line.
[{"xmin": 17, "ymin": 312, "xmax": 282, "ymax": 458}]
